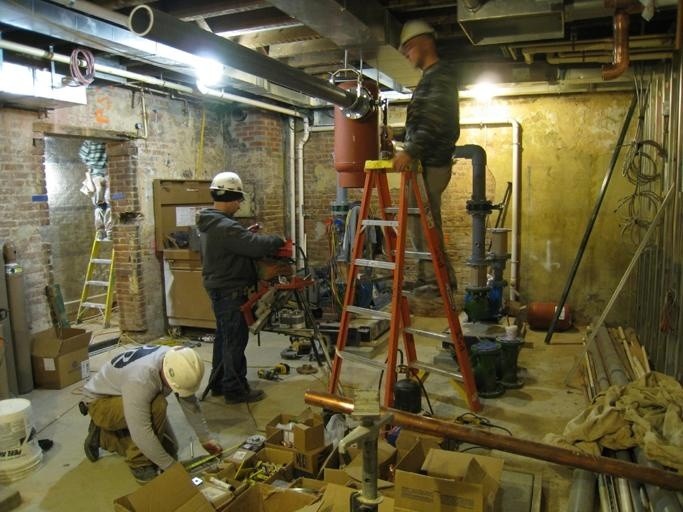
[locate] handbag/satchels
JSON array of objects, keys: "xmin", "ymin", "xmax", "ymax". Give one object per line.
[{"xmin": 79, "ymin": 171, "xmax": 97, "ymax": 199}]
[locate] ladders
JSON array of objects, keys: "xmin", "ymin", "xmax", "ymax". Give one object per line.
[
  {"xmin": 328, "ymin": 160, "xmax": 480, "ymax": 413},
  {"xmin": 76, "ymin": 231, "xmax": 115, "ymax": 329}
]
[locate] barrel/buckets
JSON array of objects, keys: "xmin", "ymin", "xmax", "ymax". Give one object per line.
[{"xmin": 1, "ymin": 397, "xmax": 45, "ymax": 484}]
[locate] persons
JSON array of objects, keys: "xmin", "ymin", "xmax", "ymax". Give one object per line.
[
  {"xmin": 79, "ymin": 341, "xmax": 222, "ymax": 488},
  {"xmin": 387, "ymin": 19, "xmax": 461, "ymax": 300},
  {"xmin": 76, "ymin": 139, "xmax": 113, "ymax": 240},
  {"xmin": 193, "ymin": 170, "xmax": 292, "ymax": 404}
]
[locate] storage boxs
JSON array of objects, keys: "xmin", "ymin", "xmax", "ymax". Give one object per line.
[
  {"xmin": 226, "ymin": 448, "xmax": 254, "ymax": 470},
  {"xmin": 265, "ymin": 412, "xmax": 326, "ymax": 449},
  {"xmin": 390, "ymin": 440, "xmax": 505, "ymax": 512},
  {"xmin": 28, "ymin": 322, "xmax": 92, "ymax": 387},
  {"xmin": 120, "ymin": 464, "xmax": 216, "ymax": 510},
  {"xmin": 382, "ymin": 432, "xmax": 421, "ymax": 481},
  {"xmin": 221, "ymin": 482, "xmax": 318, "ymax": 512},
  {"xmin": 261, "ymin": 431, "xmax": 339, "ymax": 479}
]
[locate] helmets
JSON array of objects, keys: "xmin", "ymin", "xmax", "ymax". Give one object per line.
[
  {"xmin": 208, "ymin": 171, "xmax": 246, "ymax": 194},
  {"xmin": 398, "ymin": 19, "xmax": 436, "ymax": 48},
  {"xmin": 162, "ymin": 345, "xmax": 204, "ymax": 398}
]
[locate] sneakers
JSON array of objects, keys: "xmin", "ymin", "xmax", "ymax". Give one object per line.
[
  {"xmin": 131, "ymin": 465, "xmax": 157, "ymax": 485},
  {"xmin": 210, "ymin": 388, "xmax": 264, "ymax": 404},
  {"xmin": 415, "ymin": 284, "xmax": 457, "ymax": 299},
  {"xmin": 400, "ymin": 280, "xmax": 436, "ymax": 291},
  {"xmin": 94, "ymin": 234, "xmax": 111, "ymax": 243},
  {"xmin": 83, "ymin": 420, "xmax": 99, "ymax": 461}
]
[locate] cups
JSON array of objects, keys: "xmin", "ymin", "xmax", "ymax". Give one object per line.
[{"xmin": 506, "ymin": 325, "xmax": 518, "ymax": 339}]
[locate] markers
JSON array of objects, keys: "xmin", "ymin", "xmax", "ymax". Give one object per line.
[{"xmin": 208, "ymin": 477, "xmax": 235, "ymax": 491}]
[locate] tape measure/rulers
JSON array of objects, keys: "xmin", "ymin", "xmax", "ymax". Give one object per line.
[{"xmin": 185, "ymin": 440, "xmax": 245, "ymax": 469}]
